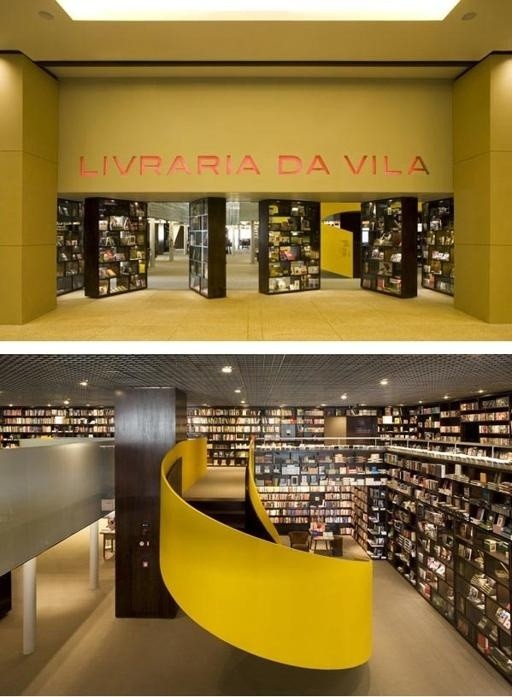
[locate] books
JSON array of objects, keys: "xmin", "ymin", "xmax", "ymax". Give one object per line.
[{"xmin": 0, "ymin": 398, "xmax": 512, "ymax": 683}]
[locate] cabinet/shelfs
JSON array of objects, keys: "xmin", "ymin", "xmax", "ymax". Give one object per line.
[
  {"xmin": 259, "ymin": 199, "xmax": 321, "ymax": 295},
  {"xmin": 422, "ymin": 199, "xmax": 454, "ymax": 296},
  {"xmin": 360, "ymin": 197, "xmax": 417, "ymax": 298},
  {"xmin": 86, "ymin": 195, "xmax": 150, "ymax": 297},
  {"xmin": 190, "ymin": 197, "xmax": 226, "ymax": 298},
  {"xmin": 57, "ymin": 197, "xmax": 85, "ymax": 297}
]
